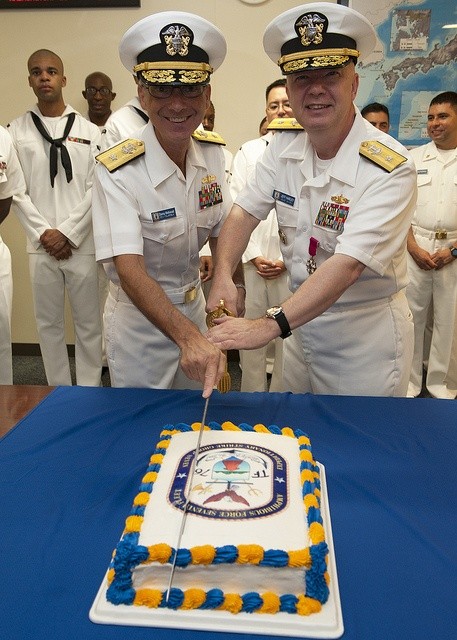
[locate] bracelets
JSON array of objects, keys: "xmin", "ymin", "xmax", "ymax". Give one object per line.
[{"xmin": 233, "ymin": 282, "xmax": 246, "ymax": 293}]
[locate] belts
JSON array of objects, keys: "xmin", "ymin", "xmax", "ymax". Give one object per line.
[
  {"xmin": 106, "ymin": 280, "xmax": 200, "ymax": 303},
  {"xmin": 412, "ymin": 227, "xmax": 457, "ymax": 238}
]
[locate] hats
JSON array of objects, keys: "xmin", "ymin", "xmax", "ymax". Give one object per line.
[
  {"xmin": 260, "ymin": 3, "xmax": 379, "ymax": 74},
  {"xmin": 116, "ymin": 10, "xmax": 228, "ymax": 87}
]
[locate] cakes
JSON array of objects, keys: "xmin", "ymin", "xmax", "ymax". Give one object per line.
[{"xmin": 89, "ymin": 422, "xmax": 345, "ymax": 640}]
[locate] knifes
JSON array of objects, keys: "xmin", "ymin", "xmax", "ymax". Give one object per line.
[{"xmin": 165, "ymin": 300, "xmax": 234, "ymax": 602}]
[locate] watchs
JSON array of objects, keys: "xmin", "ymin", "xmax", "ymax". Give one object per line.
[
  {"xmin": 265, "ymin": 305, "xmax": 292, "ymax": 339},
  {"xmin": 447, "ymin": 245, "xmax": 456, "ymax": 258}
]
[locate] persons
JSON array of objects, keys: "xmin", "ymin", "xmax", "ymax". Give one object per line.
[
  {"xmin": 257, "ymin": 112, "xmax": 272, "ymax": 140},
  {"xmin": 83, "ymin": 72, "xmax": 116, "ymax": 126},
  {"xmin": 406, "ymin": 91, "xmax": 457, "ymax": 401},
  {"xmin": 232, "ymin": 80, "xmax": 295, "ymax": 394},
  {"xmin": 0, "ymin": 123, "xmax": 27, "ymax": 383},
  {"xmin": 360, "ymin": 102, "xmax": 390, "ymax": 136},
  {"xmin": 92, "ymin": 10, "xmax": 245, "ymax": 398},
  {"xmin": 204, "ymin": 1, "xmax": 416, "ymax": 397},
  {"xmin": 11, "ymin": 48, "xmax": 102, "ymax": 386},
  {"xmin": 199, "ymin": 100, "xmax": 217, "ymax": 135}
]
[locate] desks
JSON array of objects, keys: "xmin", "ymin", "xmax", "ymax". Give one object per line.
[{"xmin": 1, "ymin": 384, "xmax": 457, "ymax": 640}]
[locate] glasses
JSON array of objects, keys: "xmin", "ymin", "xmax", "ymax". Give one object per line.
[
  {"xmin": 138, "ymin": 78, "xmax": 208, "ymax": 98},
  {"xmin": 84, "ymin": 86, "xmax": 113, "ymax": 96},
  {"xmin": 264, "ymin": 101, "xmax": 291, "ymax": 115}
]
